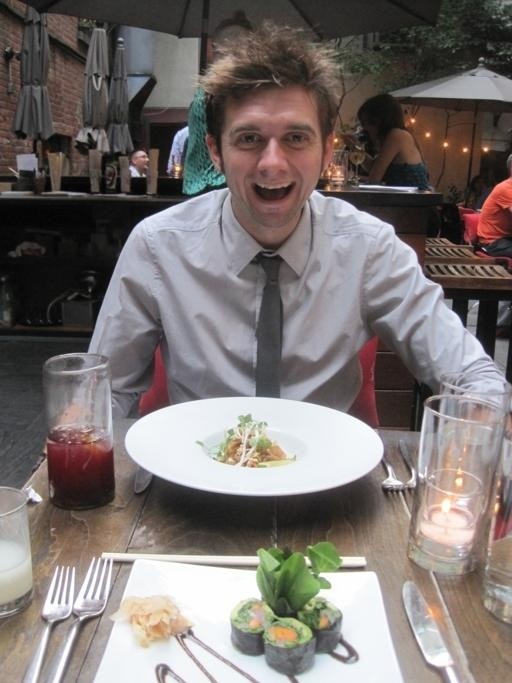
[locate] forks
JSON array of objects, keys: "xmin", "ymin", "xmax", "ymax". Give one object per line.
[
  {"xmin": 39, "ymin": 557, "xmax": 114, "ymax": 683},
  {"xmin": 399, "ymin": 439, "xmax": 419, "ymax": 491},
  {"xmin": 21, "ymin": 565, "xmax": 76, "ymax": 683},
  {"xmin": 375, "ymin": 431, "xmax": 405, "ymax": 492}
]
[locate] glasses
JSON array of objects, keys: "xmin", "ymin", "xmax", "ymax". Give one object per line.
[{"xmin": 137, "ymin": 155, "xmax": 149, "ymax": 158}]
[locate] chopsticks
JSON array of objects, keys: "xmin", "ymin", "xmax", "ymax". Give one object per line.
[{"xmin": 100, "ymin": 551, "xmax": 367, "ymax": 568}]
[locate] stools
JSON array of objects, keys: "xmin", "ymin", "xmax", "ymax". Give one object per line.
[{"xmin": 413, "ymin": 237, "xmax": 512, "ymax": 431}]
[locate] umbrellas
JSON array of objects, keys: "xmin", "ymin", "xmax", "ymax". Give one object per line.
[
  {"xmin": 17, "ymin": 0, "xmax": 444, "ymax": 82},
  {"xmin": 12, "ymin": 6, "xmax": 55, "ymax": 154},
  {"xmin": 386, "ymin": 55, "xmax": 512, "ymax": 188},
  {"xmin": 109, "ymin": 36, "xmax": 136, "ymax": 157},
  {"xmin": 73, "ymin": 19, "xmax": 110, "ymax": 154}
]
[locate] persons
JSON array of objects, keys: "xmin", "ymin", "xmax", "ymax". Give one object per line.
[
  {"xmin": 181, "ymin": 8, "xmax": 255, "ymax": 200},
  {"xmin": 475, "ymin": 152, "xmax": 512, "ymax": 337},
  {"xmin": 165, "ymin": 123, "xmax": 190, "ymax": 176},
  {"xmin": 26, "ymin": 20, "xmax": 512, "ymax": 482},
  {"xmin": 356, "ymin": 92, "xmax": 435, "ymax": 194},
  {"xmin": 128, "ymin": 148, "xmax": 150, "ymax": 178},
  {"xmin": 457, "ymin": 175, "xmax": 485, "ymax": 210}
]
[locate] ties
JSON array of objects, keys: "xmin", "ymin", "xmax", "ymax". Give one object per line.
[{"xmin": 257, "ymin": 254, "xmax": 282, "ymax": 398}]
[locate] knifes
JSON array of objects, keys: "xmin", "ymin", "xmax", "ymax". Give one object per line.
[
  {"xmin": 402, "ymin": 581, "xmax": 462, "ymax": 683},
  {"xmin": 134, "ymin": 466, "xmax": 153, "ymax": 495}
]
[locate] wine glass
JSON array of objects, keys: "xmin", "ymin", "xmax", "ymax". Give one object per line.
[{"xmin": 349, "ymin": 142, "xmax": 366, "ymax": 185}]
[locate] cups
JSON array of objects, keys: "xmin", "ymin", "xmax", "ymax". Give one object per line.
[
  {"xmin": 406, "ymin": 394, "xmax": 507, "ymax": 576},
  {"xmin": 326, "ymin": 149, "xmax": 349, "ymax": 188},
  {"xmin": 172, "ymin": 154, "xmax": 183, "ymax": 177},
  {"xmin": 436, "ymin": 371, "xmax": 512, "ymax": 570},
  {"xmin": 41, "ymin": 352, "xmax": 116, "ymax": 513},
  {"xmin": 481, "ymin": 437, "xmax": 512, "ymax": 628},
  {"xmin": 104, "ymin": 157, "xmax": 121, "ymax": 193},
  {"xmin": 1, "ymin": 485, "xmax": 35, "ymax": 621}
]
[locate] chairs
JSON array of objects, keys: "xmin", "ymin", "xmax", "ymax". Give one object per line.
[
  {"xmin": 136, "ymin": 333, "xmax": 381, "ymax": 428},
  {"xmin": 456, "ymin": 202, "xmax": 476, "ymax": 220},
  {"xmin": 462, "ymin": 212, "xmax": 512, "ymax": 272}
]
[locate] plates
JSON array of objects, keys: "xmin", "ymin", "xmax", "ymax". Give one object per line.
[
  {"xmin": 124, "ymin": 396, "xmax": 386, "ymax": 498},
  {"xmin": 92, "ymin": 558, "xmax": 405, "ymax": 683}
]
